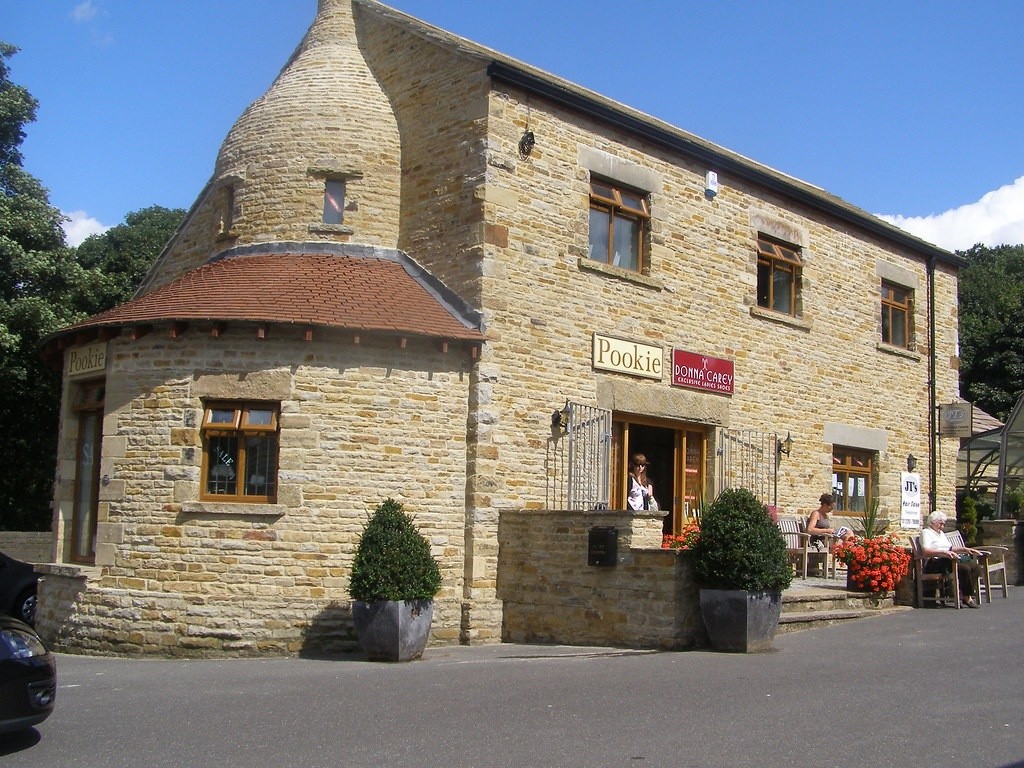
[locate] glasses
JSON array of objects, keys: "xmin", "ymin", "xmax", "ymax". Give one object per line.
[{"xmin": 636, "ymin": 464, "xmax": 645, "ymax": 467}]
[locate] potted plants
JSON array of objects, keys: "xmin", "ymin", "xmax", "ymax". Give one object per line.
[
  {"xmin": 690, "ymin": 488, "xmax": 795, "ymax": 653},
  {"xmin": 347, "ymin": 496, "xmax": 443, "ymax": 662}
]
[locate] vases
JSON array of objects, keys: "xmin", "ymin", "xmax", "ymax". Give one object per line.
[{"xmin": 845, "ymin": 563, "xmax": 889, "ymax": 593}]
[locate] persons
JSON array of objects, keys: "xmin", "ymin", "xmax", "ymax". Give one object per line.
[
  {"xmin": 627, "ymin": 453, "xmax": 658, "ymax": 511},
  {"xmin": 918, "ymin": 510, "xmax": 981, "ymax": 608},
  {"xmin": 807, "ymin": 494, "xmax": 853, "ymax": 545}
]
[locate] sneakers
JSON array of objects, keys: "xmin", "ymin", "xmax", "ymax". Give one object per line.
[{"xmin": 962, "ymin": 599, "xmax": 981, "ymax": 608}]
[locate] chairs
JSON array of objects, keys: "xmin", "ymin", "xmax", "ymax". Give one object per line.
[
  {"xmin": 801, "ymin": 515, "xmax": 842, "ymax": 579},
  {"xmin": 777, "ymin": 515, "xmax": 834, "ymax": 580},
  {"xmin": 944, "ymin": 530, "xmax": 1010, "ymax": 604},
  {"xmin": 909, "ymin": 537, "xmax": 982, "ymax": 609}
]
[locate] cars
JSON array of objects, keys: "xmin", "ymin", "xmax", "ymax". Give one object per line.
[{"xmin": 0, "ymin": 552, "xmax": 57, "ymax": 734}]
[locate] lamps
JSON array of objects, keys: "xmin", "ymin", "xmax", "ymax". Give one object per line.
[
  {"xmin": 778, "ymin": 430, "xmax": 793, "ymax": 457},
  {"xmin": 550, "ymin": 397, "xmax": 572, "ymax": 426},
  {"xmin": 908, "ymin": 454, "xmax": 917, "ymax": 471}
]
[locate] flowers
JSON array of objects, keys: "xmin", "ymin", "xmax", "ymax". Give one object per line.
[{"xmin": 831, "ymin": 501, "xmax": 911, "ymax": 590}]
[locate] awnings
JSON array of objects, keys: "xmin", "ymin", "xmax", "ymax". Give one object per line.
[{"xmin": 960, "ymin": 399, "xmax": 1005, "ymax": 434}]
